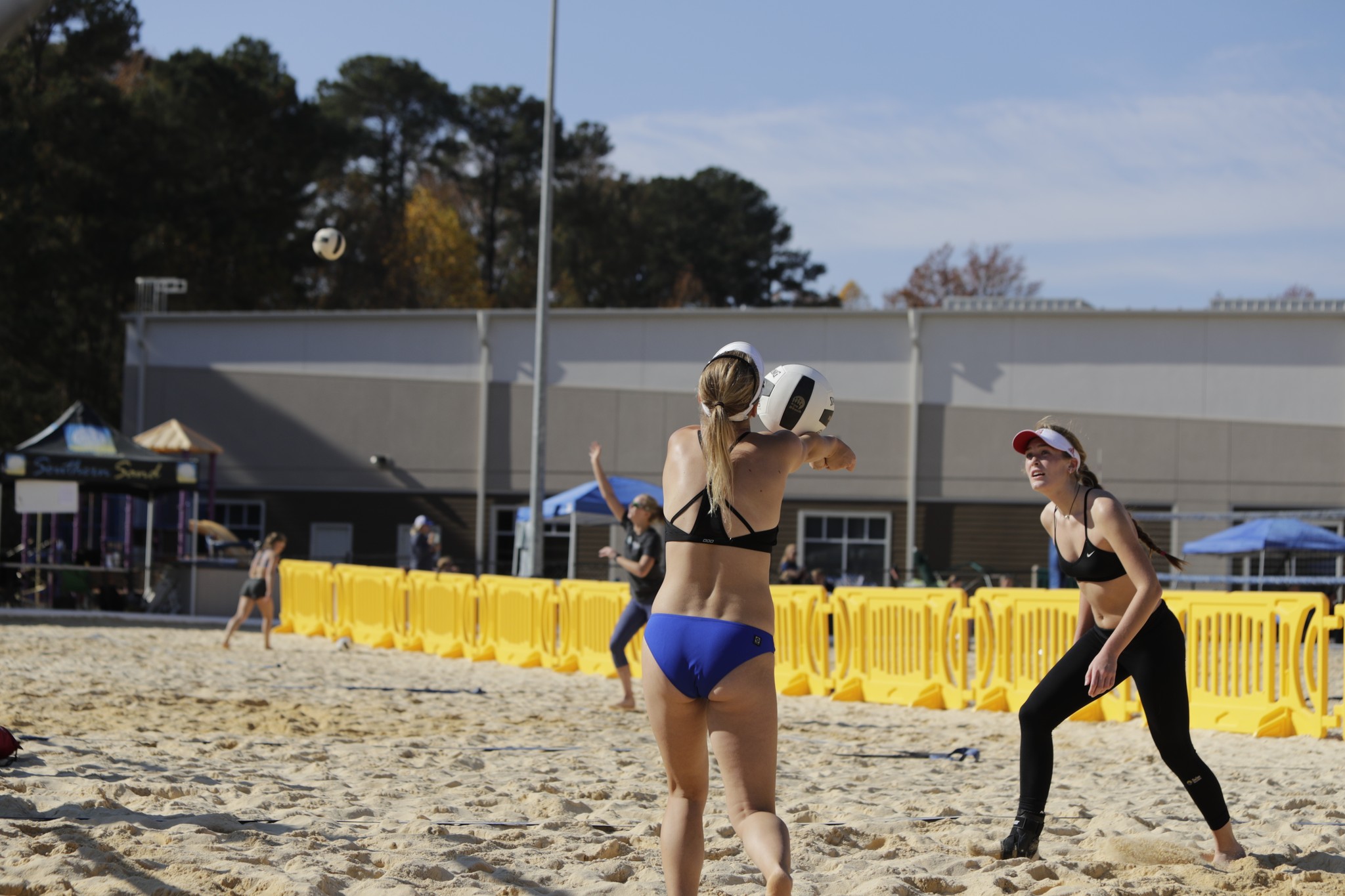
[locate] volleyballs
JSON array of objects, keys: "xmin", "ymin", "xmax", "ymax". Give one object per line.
[
  {"xmin": 756, "ymin": 364, "xmax": 835, "ymax": 438},
  {"xmin": 312, "ymin": 228, "xmax": 346, "ymax": 261}
]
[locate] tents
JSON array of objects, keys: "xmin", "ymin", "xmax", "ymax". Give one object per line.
[
  {"xmin": 542, "ymin": 473, "xmax": 663, "ymax": 578},
  {"xmin": 1181, "ymin": 515, "xmax": 1345, "ymax": 609},
  {"xmin": 0, "ymin": 416, "xmax": 222, "ymax": 608}
]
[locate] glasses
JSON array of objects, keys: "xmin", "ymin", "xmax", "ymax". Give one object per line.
[{"xmin": 631, "ymin": 502, "xmax": 643, "ymax": 509}]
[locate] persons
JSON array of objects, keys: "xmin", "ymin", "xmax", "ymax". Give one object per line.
[
  {"xmin": 408, "ymin": 514, "xmax": 458, "ymax": 571},
  {"xmin": 778, "ymin": 541, "xmax": 835, "ymax": 591},
  {"xmin": 971, "ymin": 424, "xmax": 1247, "ymax": 865},
  {"xmin": 632, "ymin": 339, "xmax": 860, "ymax": 896},
  {"xmin": 946, "ymin": 573, "xmax": 962, "ymax": 587},
  {"xmin": 223, "ymin": 532, "xmax": 287, "ymax": 649},
  {"xmin": 585, "ymin": 437, "xmax": 662, "ymax": 709}
]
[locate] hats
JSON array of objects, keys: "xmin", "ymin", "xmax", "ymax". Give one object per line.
[
  {"xmin": 701, "ymin": 341, "xmax": 764, "ymax": 422},
  {"xmin": 1012, "ymin": 428, "xmax": 1081, "ymax": 472},
  {"xmin": 414, "ymin": 515, "xmax": 434, "ymax": 530}
]
